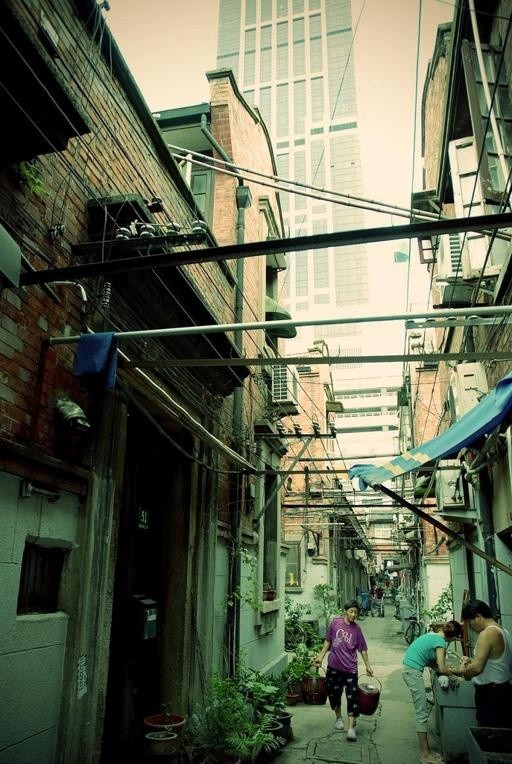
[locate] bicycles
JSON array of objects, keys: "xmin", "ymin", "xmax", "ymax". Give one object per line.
[
  {"xmin": 285, "ymin": 614, "xmax": 305, "ymax": 653},
  {"xmin": 405, "ymin": 613, "xmax": 420, "ymax": 644}
]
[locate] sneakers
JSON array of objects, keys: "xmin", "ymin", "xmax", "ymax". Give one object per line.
[
  {"xmin": 346, "ymin": 728, "xmax": 358, "ymax": 742},
  {"xmin": 419, "ymin": 749, "xmax": 445, "ymax": 763},
  {"xmin": 334, "ymin": 716, "xmax": 345, "ymax": 730}
]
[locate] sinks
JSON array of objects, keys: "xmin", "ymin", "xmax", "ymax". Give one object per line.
[{"xmin": 468, "ymin": 726, "xmax": 512, "ymax": 753}]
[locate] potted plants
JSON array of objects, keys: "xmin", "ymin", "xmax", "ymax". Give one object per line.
[{"xmin": 143, "ymin": 643, "xmax": 308, "ymax": 763}]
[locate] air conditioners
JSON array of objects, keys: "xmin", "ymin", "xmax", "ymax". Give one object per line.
[
  {"xmin": 446, "ymin": 360, "xmax": 488, "ymax": 430},
  {"xmin": 436, "ymin": 133, "xmax": 504, "ymax": 306},
  {"xmin": 435, "ymin": 458, "xmax": 461, "ymax": 511},
  {"xmin": 272, "ymin": 360, "xmax": 300, "ymax": 405}
]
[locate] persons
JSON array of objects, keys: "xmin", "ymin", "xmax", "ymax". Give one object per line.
[
  {"xmin": 400, "ymin": 620, "xmax": 462, "ymax": 764},
  {"xmin": 459, "ymin": 599, "xmax": 512, "ymax": 729},
  {"xmin": 312, "ymin": 600, "xmax": 373, "ymax": 742},
  {"xmin": 374, "ymin": 568, "xmax": 391, "ymax": 617}
]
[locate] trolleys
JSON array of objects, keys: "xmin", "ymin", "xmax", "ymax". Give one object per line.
[{"xmin": 371, "ymin": 596, "xmax": 384, "ymax": 617}]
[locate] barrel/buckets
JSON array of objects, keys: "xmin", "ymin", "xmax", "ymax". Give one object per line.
[
  {"xmin": 302, "ymin": 665, "xmax": 328, "ymax": 705},
  {"xmin": 357, "ymin": 673, "xmax": 382, "ymax": 716}
]
